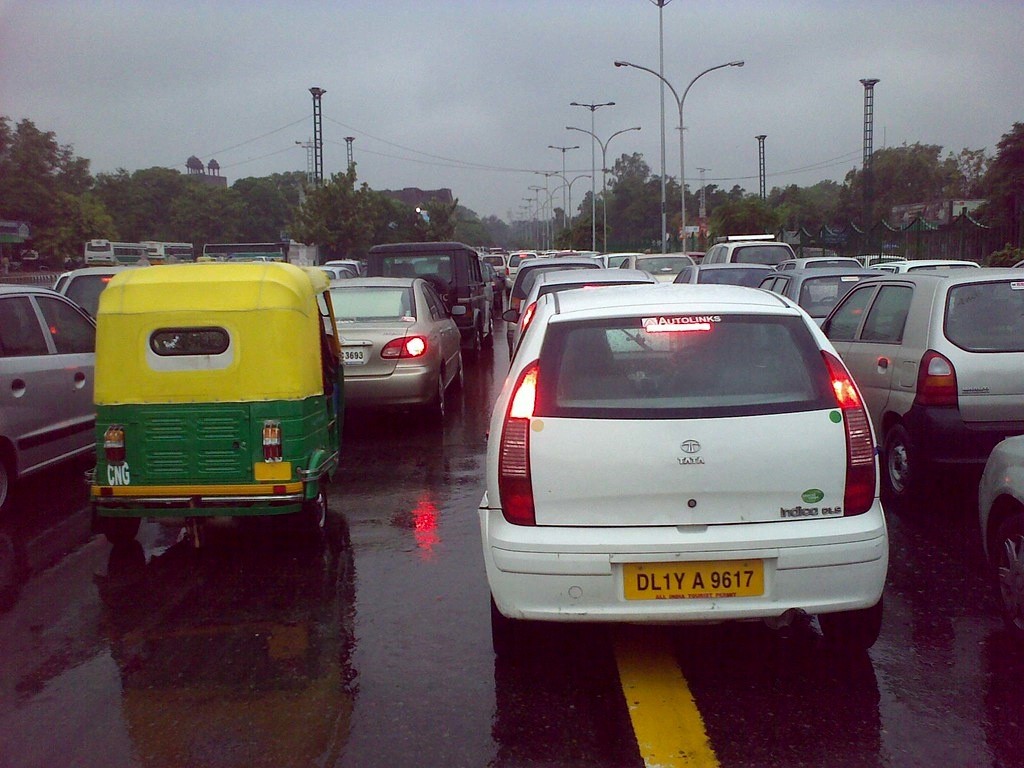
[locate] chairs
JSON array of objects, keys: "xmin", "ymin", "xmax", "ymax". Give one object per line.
[
  {"xmin": 437, "ymin": 260, "xmax": 454, "ymax": 284},
  {"xmin": 390, "ymin": 263, "xmax": 416, "ymax": 278},
  {"xmin": 560, "ymin": 328, "xmax": 618, "ymax": 378}
]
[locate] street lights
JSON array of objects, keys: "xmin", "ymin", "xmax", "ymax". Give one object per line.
[
  {"xmin": 547, "ymin": 145, "xmax": 580, "ymax": 229},
  {"xmin": 569, "ymin": 102, "xmax": 616, "ymax": 251},
  {"xmin": 565, "ymin": 124, "xmax": 642, "ymax": 255},
  {"xmin": 613, "ymin": 58, "xmax": 747, "ymax": 252},
  {"xmin": 518, "ymin": 184, "xmax": 564, "ymax": 251}
]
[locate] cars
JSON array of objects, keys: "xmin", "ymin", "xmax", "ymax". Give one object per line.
[
  {"xmin": 0, "ymin": 281, "xmax": 97, "ymax": 517},
  {"xmin": 478, "ymin": 284, "xmax": 891, "ymax": 658},
  {"xmin": 328, "ymin": 277, "xmax": 466, "ymax": 438},
  {"xmin": 315, "ymin": 234, "xmax": 982, "ymax": 372},
  {"xmin": 977, "ymin": 438, "xmax": 1024, "ymax": 640},
  {"xmin": 53, "ymin": 268, "xmax": 131, "ymax": 323}
]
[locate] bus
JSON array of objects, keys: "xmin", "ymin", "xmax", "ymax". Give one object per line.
[
  {"xmin": 202, "ymin": 243, "xmax": 287, "ymax": 263},
  {"xmin": 83, "ymin": 237, "xmax": 150, "ymax": 268},
  {"xmin": 138, "ymin": 240, "xmax": 199, "ymax": 267}
]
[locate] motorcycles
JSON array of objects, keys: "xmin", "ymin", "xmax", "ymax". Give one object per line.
[{"xmin": 89, "ymin": 260, "xmax": 344, "ymax": 550}]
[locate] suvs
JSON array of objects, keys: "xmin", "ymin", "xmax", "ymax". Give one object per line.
[{"xmin": 819, "ymin": 269, "xmax": 1024, "ymax": 522}]
